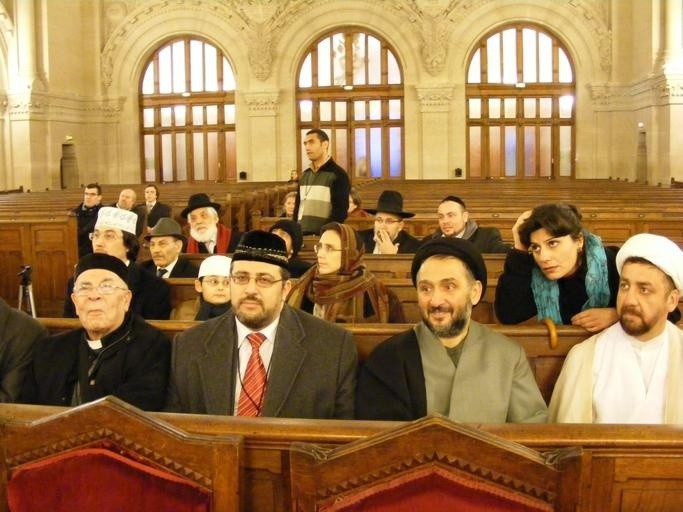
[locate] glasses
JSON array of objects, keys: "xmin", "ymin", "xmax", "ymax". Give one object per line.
[
  {"xmin": 73, "ymin": 282, "xmax": 130, "ymax": 295},
  {"xmin": 317, "ymin": 242, "xmax": 345, "ymax": 253},
  {"xmin": 202, "ymin": 278, "xmax": 230, "ymax": 287},
  {"xmin": 229, "ymin": 270, "xmax": 285, "ymax": 288},
  {"xmin": 88, "ymin": 233, "xmax": 124, "ymax": 242},
  {"xmin": 374, "ymin": 217, "xmax": 400, "ymax": 225}
]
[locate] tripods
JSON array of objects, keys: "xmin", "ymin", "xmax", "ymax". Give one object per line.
[{"xmin": 18, "ymin": 286, "xmax": 37, "ymax": 319}]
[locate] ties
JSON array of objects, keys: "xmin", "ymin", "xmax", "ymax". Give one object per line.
[
  {"xmin": 147, "ymin": 203, "xmax": 152, "ymax": 215},
  {"xmin": 236, "ymin": 332, "xmax": 268, "ymax": 417},
  {"xmin": 158, "ymin": 268, "xmax": 168, "ymax": 278}
]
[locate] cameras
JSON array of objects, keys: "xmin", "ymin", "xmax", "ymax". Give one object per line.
[{"xmin": 14, "ymin": 264, "xmax": 33, "ymax": 286}]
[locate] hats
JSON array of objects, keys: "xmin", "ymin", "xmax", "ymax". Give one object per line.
[
  {"xmin": 180, "ymin": 193, "xmax": 222, "ymax": 218},
  {"xmin": 269, "ymin": 219, "xmax": 304, "ymax": 257},
  {"xmin": 198, "ymin": 255, "xmax": 232, "ymax": 279},
  {"xmin": 94, "ymin": 207, "xmax": 138, "ymax": 236},
  {"xmin": 442, "ymin": 196, "xmax": 465, "ymax": 209},
  {"xmin": 231, "ymin": 230, "xmax": 291, "ymax": 273},
  {"xmin": 72, "ymin": 252, "xmax": 132, "ymax": 290},
  {"xmin": 143, "ymin": 217, "xmax": 188, "ymax": 253},
  {"xmin": 363, "ymin": 190, "xmax": 415, "ymax": 218}
]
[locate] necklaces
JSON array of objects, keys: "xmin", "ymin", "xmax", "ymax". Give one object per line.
[{"xmin": 238, "ymin": 345, "xmax": 274, "ymax": 416}]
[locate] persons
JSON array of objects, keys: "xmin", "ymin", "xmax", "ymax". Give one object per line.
[
  {"xmin": 32, "ymin": 254, "xmax": 170, "ymax": 414},
  {"xmin": 288, "ymin": 171, "xmax": 299, "ymax": 182},
  {"xmin": 423, "ymin": 196, "xmax": 512, "ymax": 254},
  {"xmin": 356, "ymin": 237, "xmax": 547, "ymax": 424},
  {"xmin": 71, "ymin": 184, "xmax": 363, "ymax": 321},
  {"xmin": 288, "ymin": 222, "xmax": 404, "ymax": 323},
  {"xmin": 494, "ymin": 204, "xmax": 682, "ymax": 334},
  {"xmin": 356, "ymin": 190, "xmax": 418, "ymax": 255},
  {"xmin": 550, "ymin": 234, "xmax": 683, "ymax": 425},
  {"xmin": 174, "ymin": 229, "xmax": 359, "ymax": 421},
  {"xmin": 293, "ymin": 128, "xmax": 350, "ymax": 235},
  {"xmin": 0, "ymin": 296, "xmax": 48, "ymax": 402}
]
